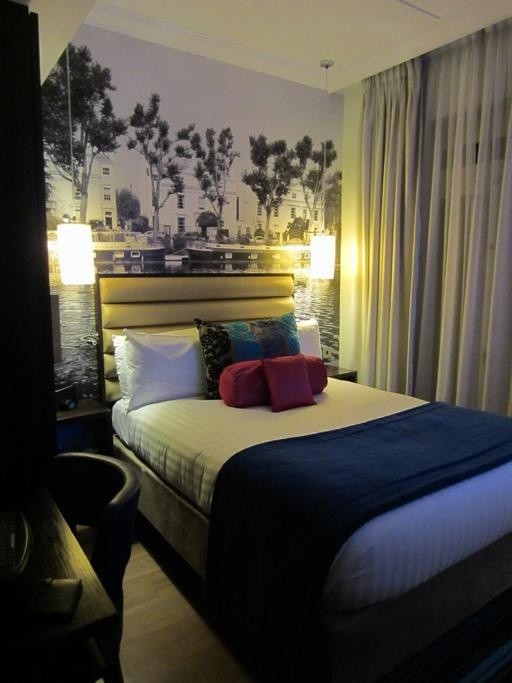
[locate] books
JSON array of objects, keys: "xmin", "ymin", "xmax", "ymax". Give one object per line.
[{"xmin": 16, "ymin": 577, "xmax": 85, "ymax": 623}]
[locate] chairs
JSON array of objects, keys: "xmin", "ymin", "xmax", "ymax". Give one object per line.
[{"xmin": 41, "ymin": 449, "xmax": 141, "ymax": 682}]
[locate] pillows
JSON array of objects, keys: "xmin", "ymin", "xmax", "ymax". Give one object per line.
[{"xmin": 110, "ymin": 314, "xmax": 328, "ymax": 413}]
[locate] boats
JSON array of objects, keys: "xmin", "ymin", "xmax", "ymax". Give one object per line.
[
  {"xmin": 187, "ymin": 241, "xmax": 312, "ymax": 262},
  {"xmin": 46, "ymin": 227, "xmax": 166, "ymax": 262}
]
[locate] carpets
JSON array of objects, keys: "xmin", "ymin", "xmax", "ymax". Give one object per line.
[{"xmin": 379, "ymin": 585, "xmax": 511, "ymax": 682}]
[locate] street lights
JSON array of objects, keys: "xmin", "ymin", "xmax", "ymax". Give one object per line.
[{"xmin": 321, "ymin": 141, "xmax": 326, "ymax": 233}]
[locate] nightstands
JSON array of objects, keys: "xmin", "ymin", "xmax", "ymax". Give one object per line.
[{"xmin": 56, "ymin": 398, "xmax": 112, "ymax": 456}]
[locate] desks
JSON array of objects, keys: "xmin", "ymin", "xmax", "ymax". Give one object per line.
[{"xmin": 1, "ymin": 489, "xmax": 120, "ymax": 683}]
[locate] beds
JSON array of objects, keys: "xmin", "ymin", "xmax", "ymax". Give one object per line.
[{"xmin": 95, "ymin": 273, "xmax": 511, "ymax": 611}]
